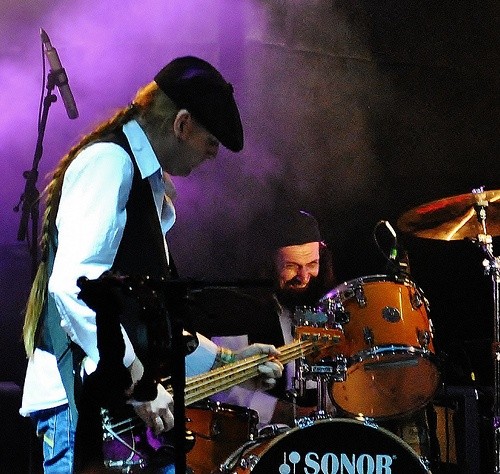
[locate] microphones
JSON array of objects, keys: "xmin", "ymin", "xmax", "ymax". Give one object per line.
[
  {"xmin": 387, "ymin": 243, "xmax": 397, "ymax": 273},
  {"xmin": 40, "ymin": 30, "xmax": 79, "ymax": 121}
]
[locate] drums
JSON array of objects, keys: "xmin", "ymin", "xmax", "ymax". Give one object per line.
[
  {"xmin": 184, "ymin": 400, "xmax": 259, "ymax": 474},
  {"xmin": 223, "ymin": 418, "xmax": 431, "ymax": 474},
  {"xmin": 395, "ymin": 393, "xmax": 466, "ymax": 466},
  {"xmin": 305, "ymin": 275, "xmax": 442, "ymax": 421}
]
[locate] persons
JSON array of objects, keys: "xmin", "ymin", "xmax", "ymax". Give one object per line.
[
  {"xmin": 233, "ymin": 207, "xmax": 342, "ymax": 412},
  {"xmin": 19, "ymin": 55, "xmax": 284, "ymax": 474}
]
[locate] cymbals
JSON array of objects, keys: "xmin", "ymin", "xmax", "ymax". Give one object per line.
[{"xmin": 397, "ymin": 190, "xmax": 500, "ymax": 241}]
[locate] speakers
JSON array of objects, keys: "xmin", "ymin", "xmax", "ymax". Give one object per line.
[
  {"xmin": 176, "ymin": 398, "xmax": 257, "ymax": 474},
  {"xmin": 380, "ymin": 386, "xmax": 483, "ymax": 474}
]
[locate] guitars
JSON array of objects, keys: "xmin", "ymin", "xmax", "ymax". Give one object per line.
[{"xmin": 100, "ymin": 305, "xmax": 345, "ymax": 472}]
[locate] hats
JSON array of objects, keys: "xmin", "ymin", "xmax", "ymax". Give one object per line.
[
  {"xmin": 152, "ymin": 54, "xmax": 245, "ymax": 154},
  {"xmin": 266, "ymin": 209, "xmax": 324, "ymax": 252}
]
